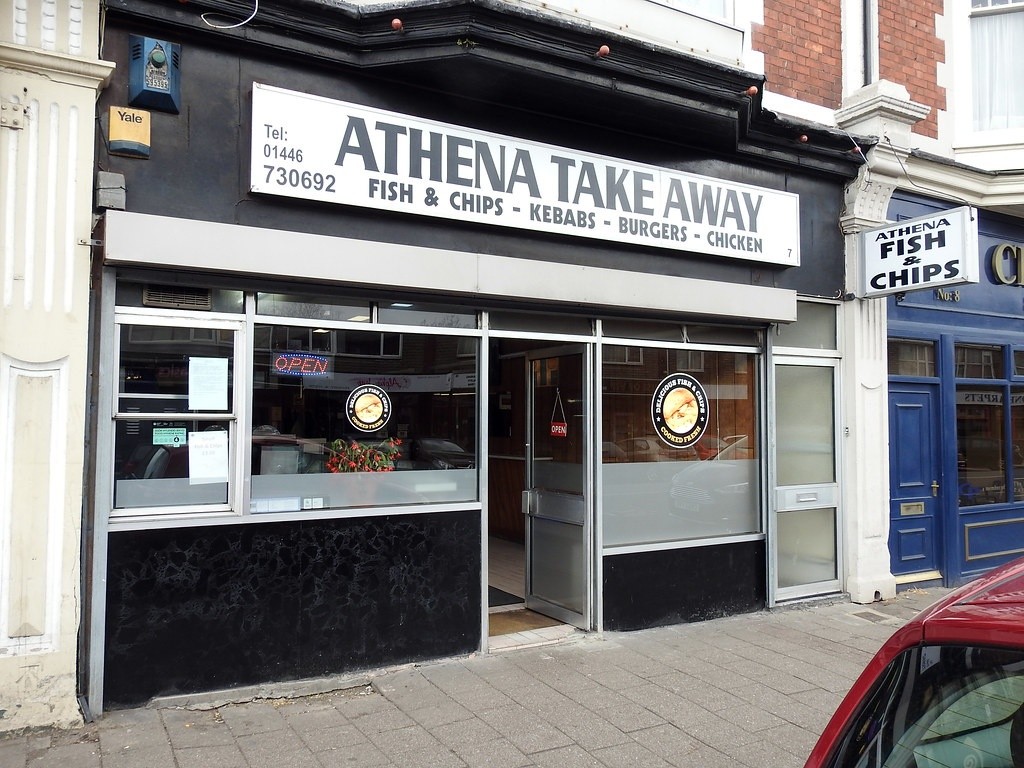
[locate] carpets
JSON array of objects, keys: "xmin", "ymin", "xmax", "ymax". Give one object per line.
[
  {"xmin": 489, "ymin": 586, "xmax": 525, "ymax": 607},
  {"xmin": 489, "ymin": 609, "xmax": 565, "ymax": 637}
]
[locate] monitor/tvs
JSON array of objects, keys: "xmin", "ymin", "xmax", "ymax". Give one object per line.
[{"xmin": 116, "ymin": 393, "xmax": 196, "ymax": 481}]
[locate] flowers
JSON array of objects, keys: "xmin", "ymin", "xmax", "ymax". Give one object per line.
[{"xmin": 328, "ymin": 438, "xmax": 402, "ymax": 474}]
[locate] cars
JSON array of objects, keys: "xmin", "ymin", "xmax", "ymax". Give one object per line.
[
  {"xmin": 601, "ymin": 431, "xmax": 748, "ymax": 464},
  {"xmin": 115, "ymin": 434, "xmax": 476, "ymax": 480},
  {"xmin": 803, "ymin": 555, "xmax": 1024, "ymax": 768}
]
